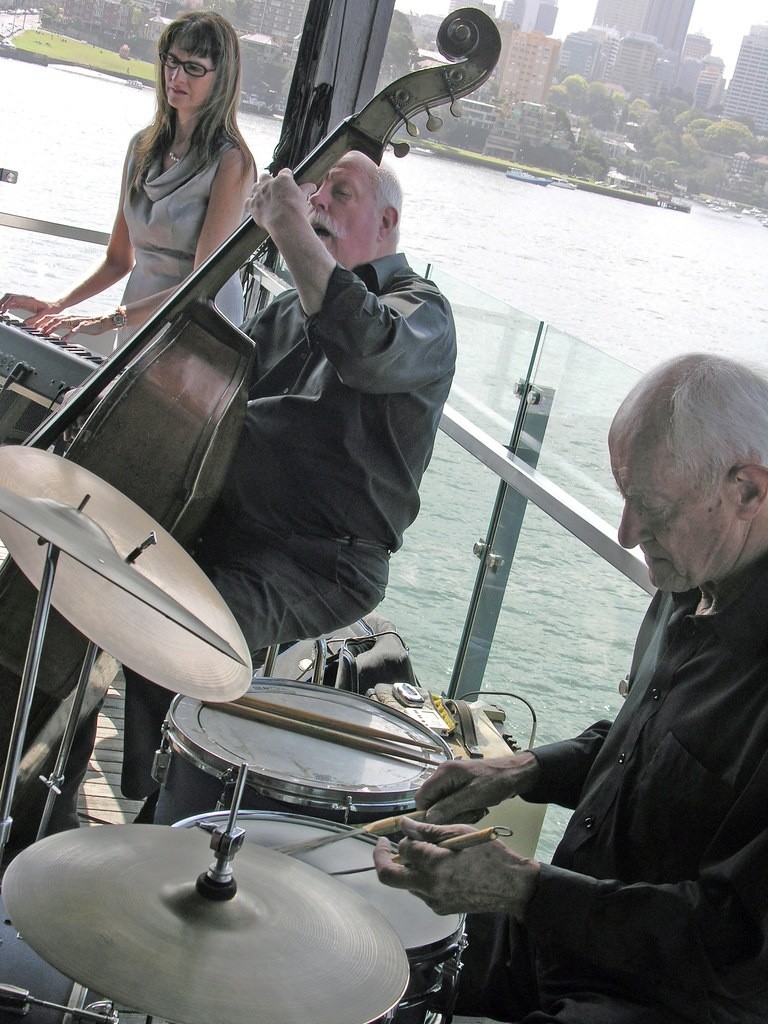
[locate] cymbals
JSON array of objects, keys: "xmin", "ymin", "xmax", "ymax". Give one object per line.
[
  {"xmin": 1, "ymin": 822, "xmax": 411, "ymax": 1024},
  {"xmin": 0, "ymin": 441, "xmax": 255, "ymax": 704}
]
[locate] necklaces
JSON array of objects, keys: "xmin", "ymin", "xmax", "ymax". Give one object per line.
[{"xmin": 168, "ymin": 152, "xmax": 180, "ymax": 162}]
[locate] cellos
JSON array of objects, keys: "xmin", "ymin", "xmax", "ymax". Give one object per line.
[{"xmin": 0, "ymin": 5, "xmax": 506, "ymax": 702}]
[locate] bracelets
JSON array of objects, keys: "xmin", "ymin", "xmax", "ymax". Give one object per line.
[{"xmin": 118, "ymin": 306, "xmax": 128, "ymax": 326}]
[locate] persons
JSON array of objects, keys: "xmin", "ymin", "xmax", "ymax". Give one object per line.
[
  {"xmin": 371, "ymin": 353, "xmax": 768, "ymax": 1024},
  {"xmin": 0, "ymin": 152, "xmax": 459, "ymax": 855},
  {"xmin": 0, "ymin": 10, "xmax": 259, "ymax": 356}
]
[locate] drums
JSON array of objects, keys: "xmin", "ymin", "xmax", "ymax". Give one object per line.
[{"xmin": 147, "ymin": 674, "xmax": 471, "ymax": 1024}]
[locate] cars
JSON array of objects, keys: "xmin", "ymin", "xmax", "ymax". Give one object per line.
[
  {"xmin": 610, "ymin": 185, "xmax": 622, "ymax": 190},
  {"xmin": 595, "ymin": 180, "xmax": 607, "ymax": 186},
  {"xmin": 7, "ymin": 8, "xmax": 38, "ymax": 15},
  {"xmin": 576, "ymin": 176, "xmax": 589, "ymax": 182}
]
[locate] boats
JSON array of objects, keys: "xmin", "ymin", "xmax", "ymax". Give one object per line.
[
  {"xmin": 243, "ymin": 97, "xmax": 274, "ymax": 117},
  {"xmin": 407, "ymin": 147, "xmax": 433, "ymax": 157},
  {"xmin": 552, "ymin": 177, "xmax": 576, "ymax": 189},
  {"xmin": 125, "ymin": 79, "xmax": 144, "ymax": 90},
  {"xmin": 504, "ymin": 167, "xmax": 552, "ymax": 187}
]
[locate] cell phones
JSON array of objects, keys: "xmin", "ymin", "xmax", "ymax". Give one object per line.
[{"xmin": 393, "ymin": 683, "xmax": 425, "ymax": 709}]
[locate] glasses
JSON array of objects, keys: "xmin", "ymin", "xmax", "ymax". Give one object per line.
[{"xmin": 159, "ymin": 50, "xmax": 217, "ymax": 77}]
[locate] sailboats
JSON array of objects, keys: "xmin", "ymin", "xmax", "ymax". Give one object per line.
[{"xmin": 683, "ymin": 182, "xmax": 768, "ymax": 226}]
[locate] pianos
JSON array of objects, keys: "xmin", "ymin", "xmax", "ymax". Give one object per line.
[{"xmin": 0, "ymin": 308, "xmax": 130, "ymax": 457}]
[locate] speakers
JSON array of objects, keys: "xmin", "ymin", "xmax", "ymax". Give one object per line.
[{"xmin": 366, "ymin": 688, "xmax": 548, "ymax": 859}]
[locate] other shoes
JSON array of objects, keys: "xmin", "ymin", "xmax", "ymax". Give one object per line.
[{"xmin": 133, "ymin": 788, "xmax": 163, "ymax": 824}]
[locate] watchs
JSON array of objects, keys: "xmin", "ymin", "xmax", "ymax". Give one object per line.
[{"xmin": 114, "ymin": 310, "xmax": 124, "ymax": 327}]
